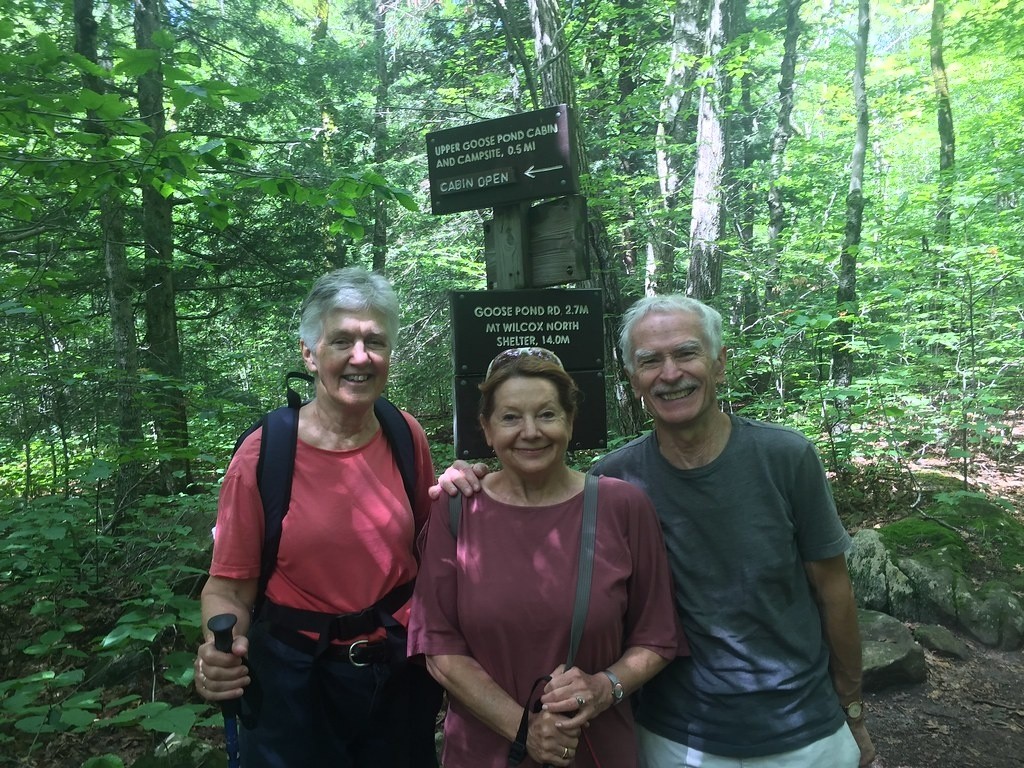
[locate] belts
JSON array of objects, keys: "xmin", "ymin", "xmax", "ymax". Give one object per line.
[{"xmin": 267, "ymin": 623, "xmax": 385, "ymax": 666}]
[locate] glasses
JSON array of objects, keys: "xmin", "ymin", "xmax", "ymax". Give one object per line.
[{"xmin": 484, "ymin": 346, "xmax": 565, "ymax": 381}]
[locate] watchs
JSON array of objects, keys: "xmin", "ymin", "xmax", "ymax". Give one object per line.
[
  {"xmin": 601, "ymin": 669, "xmax": 624, "ymax": 709},
  {"xmin": 840, "ymin": 698, "xmax": 866, "ymax": 724}
]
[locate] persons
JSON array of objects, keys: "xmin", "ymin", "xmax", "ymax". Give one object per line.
[
  {"xmin": 194, "ymin": 265, "xmax": 438, "ymax": 767},
  {"xmin": 406, "ymin": 346, "xmax": 676, "ymax": 767},
  {"xmin": 588, "ymin": 294, "xmax": 876, "ymax": 768}
]
